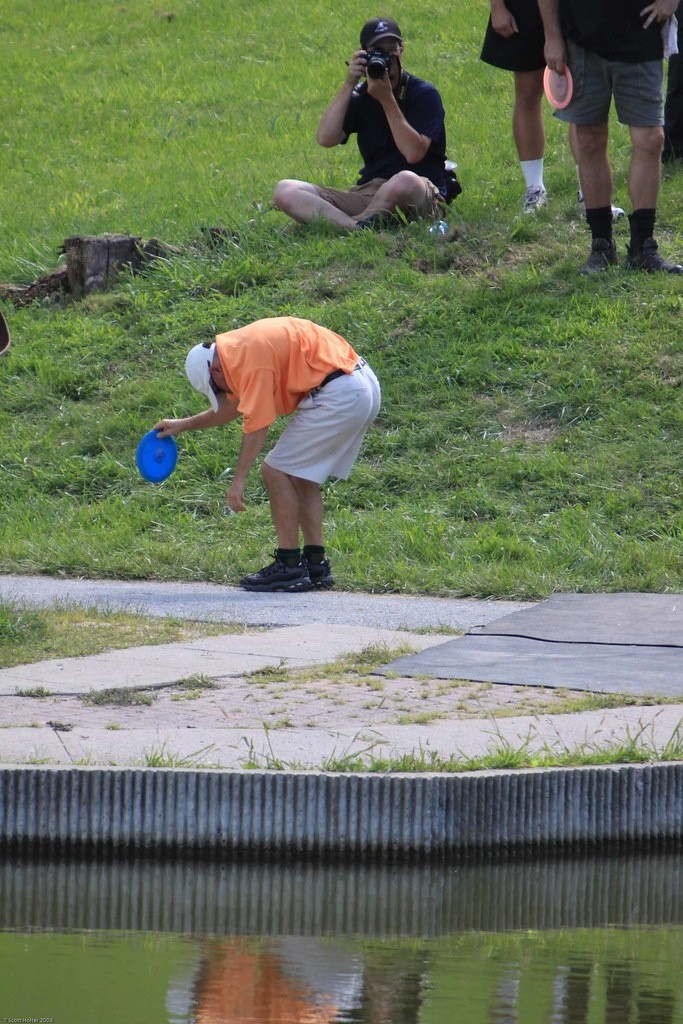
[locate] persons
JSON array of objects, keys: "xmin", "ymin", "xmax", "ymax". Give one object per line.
[
  {"xmin": 273, "ymin": 17, "xmax": 464, "ymax": 236},
  {"xmin": 152, "ymin": 316, "xmax": 383, "ymax": 594},
  {"xmin": 480, "ymin": 0, "xmax": 683, "ymax": 276}
]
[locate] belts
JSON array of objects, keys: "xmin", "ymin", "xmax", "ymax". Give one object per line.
[{"xmin": 315, "ymin": 359, "xmax": 366, "ymax": 394}]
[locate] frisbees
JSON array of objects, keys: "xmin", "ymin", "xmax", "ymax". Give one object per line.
[
  {"xmin": 543, "ymin": 63, "xmax": 573, "ymax": 111},
  {"xmin": 136, "ymin": 429, "xmax": 178, "ymax": 483}
]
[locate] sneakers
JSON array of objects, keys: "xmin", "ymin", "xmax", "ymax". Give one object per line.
[
  {"xmin": 522, "ymin": 188, "xmax": 548, "ymax": 213},
  {"xmin": 307, "ymin": 557, "xmax": 334, "ymax": 588},
  {"xmin": 578, "ymin": 238, "xmax": 619, "ymax": 277},
  {"xmin": 625, "ymin": 241, "xmax": 683, "ymax": 275},
  {"xmin": 576, "ymin": 190, "xmax": 625, "ymax": 223},
  {"xmin": 238, "ymin": 551, "xmax": 311, "ymax": 592}
]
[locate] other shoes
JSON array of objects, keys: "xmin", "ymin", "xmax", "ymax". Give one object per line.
[{"xmin": 661, "ymin": 135, "xmax": 683, "ymax": 164}]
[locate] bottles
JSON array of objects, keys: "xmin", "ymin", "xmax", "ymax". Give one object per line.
[
  {"xmin": 611, "ymin": 203, "xmax": 625, "ymax": 222},
  {"xmin": 429, "ymin": 220, "xmax": 448, "ymax": 236}
]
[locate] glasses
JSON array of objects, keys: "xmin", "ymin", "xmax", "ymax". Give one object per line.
[{"xmin": 205, "ymin": 360, "xmax": 225, "ymax": 394}]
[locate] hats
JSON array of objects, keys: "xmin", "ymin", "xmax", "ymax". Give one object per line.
[
  {"xmin": 360, "ymin": 18, "xmax": 402, "ymax": 50},
  {"xmin": 183, "ymin": 342, "xmax": 218, "ymax": 412}
]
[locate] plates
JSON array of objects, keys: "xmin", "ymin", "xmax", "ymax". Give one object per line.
[
  {"xmin": 136, "ymin": 430, "xmax": 177, "ymax": 483},
  {"xmin": 543, "ymin": 64, "xmax": 573, "ymax": 109}
]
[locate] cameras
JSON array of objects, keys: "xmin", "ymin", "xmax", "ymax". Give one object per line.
[{"xmin": 363, "ymin": 49, "xmax": 392, "ymax": 79}]
